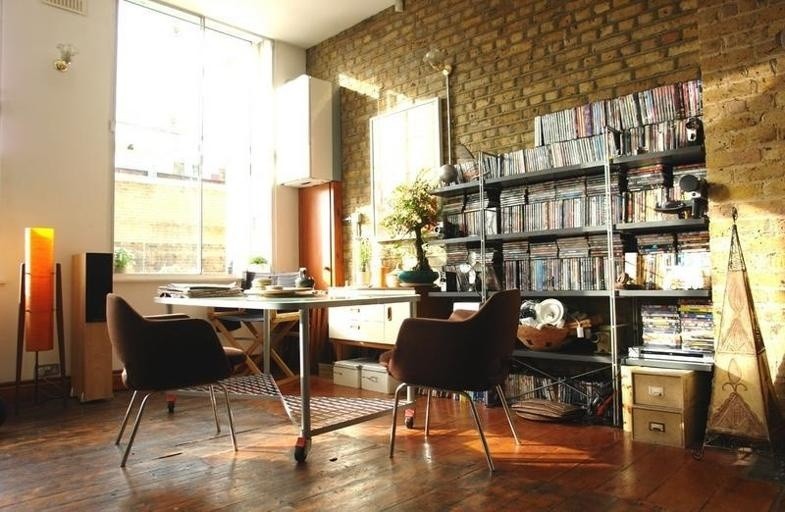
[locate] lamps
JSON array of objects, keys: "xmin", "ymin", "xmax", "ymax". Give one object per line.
[
  {"xmin": 13, "ymin": 225, "xmax": 70, "ymax": 413},
  {"xmin": 53, "ymin": 42, "xmax": 81, "ymax": 72},
  {"xmin": 422, "ymin": 50, "xmax": 452, "ymax": 77}
]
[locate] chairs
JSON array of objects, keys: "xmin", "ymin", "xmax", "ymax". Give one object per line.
[
  {"xmin": 105, "ymin": 293, "xmax": 247, "ymax": 467},
  {"xmin": 378, "ymin": 289, "xmax": 521, "ymax": 473}
]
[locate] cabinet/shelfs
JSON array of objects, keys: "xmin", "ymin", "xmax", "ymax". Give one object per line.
[
  {"xmin": 327, "ymin": 286, "xmax": 441, "ymax": 362},
  {"xmin": 427, "ymin": 123, "xmax": 712, "ymax": 426},
  {"xmin": 208, "ymin": 311, "xmax": 298, "ymax": 387}
]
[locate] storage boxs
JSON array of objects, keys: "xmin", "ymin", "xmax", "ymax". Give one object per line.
[
  {"xmin": 629, "ymin": 367, "xmax": 714, "ymax": 449},
  {"xmin": 333, "ymin": 358, "xmax": 404, "ymax": 394}
]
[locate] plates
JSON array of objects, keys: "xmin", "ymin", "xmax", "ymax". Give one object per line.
[
  {"xmin": 266, "ymin": 286, "xmax": 311, "ymax": 291},
  {"xmin": 400, "ymin": 282, "xmax": 435, "ymax": 287},
  {"xmin": 259, "ymin": 291, "xmax": 314, "ymax": 297}
]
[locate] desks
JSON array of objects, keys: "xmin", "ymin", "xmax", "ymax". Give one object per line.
[{"xmin": 153, "ymin": 294, "xmax": 422, "ymax": 463}]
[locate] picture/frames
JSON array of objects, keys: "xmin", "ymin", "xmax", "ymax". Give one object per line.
[{"xmin": 368, "ymin": 96, "xmax": 442, "ymax": 242}]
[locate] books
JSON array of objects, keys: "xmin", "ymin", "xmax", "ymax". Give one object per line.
[
  {"xmin": 502, "ymin": 235, "xmax": 623, "ymax": 289},
  {"xmin": 418, "ymin": 386, "xmax": 498, "ymax": 406},
  {"xmin": 490, "ymin": 148, "xmax": 534, "ymax": 176},
  {"xmin": 615, "ymin": 80, "xmax": 704, "ymax": 156},
  {"xmin": 444, "ymin": 191, "xmax": 500, "ymax": 236},
  {"xmin": 641, "ymin": 305, "xmax": 682, "ymax": 348},
  {"xmin": 452, "ymin": 159, "xmax": 481, "ymax": 183},
  {"xmin": 494, "ymin": 172, "xmax": 620, "ymax": 233},
  {"xmin": 425, "ymin": 243, "xmax": 501, "ymax": 290},
  {"xmin": 621, "ymin": 162, "xmax": 708, "ymax": 222},
  {"xmin": 625, "ymin": 232, "xmax": 711, "ymax": 289},
  {"xmin": 505, "ymin": 364, "xmax": 612, "ymax": 419},
  {"xmin": 157, "ymin": 283, "xmax": 246, "ymax": 298},
  {"xmin": 679, "ymin": 305, "xmax": 715, "ymax": 351},
  {"xmin": 535, "ymin": 101, "xmax": 614, "ymax": 170}
]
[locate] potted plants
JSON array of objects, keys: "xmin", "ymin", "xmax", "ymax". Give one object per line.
[
  {"xmin": 113, "ymin": 248, "xmax": 134, "ymax": 273},
  {"xmin": 358, "ymin": 237, "xmax": 371, "ymax": 286},
  {"xmin": 378, "ymin": 167, "xmax": 439, "ymax": 287},
  {"xmin": 249, "ymin": 256, "xmax": 269, "ymax": 273}
]
